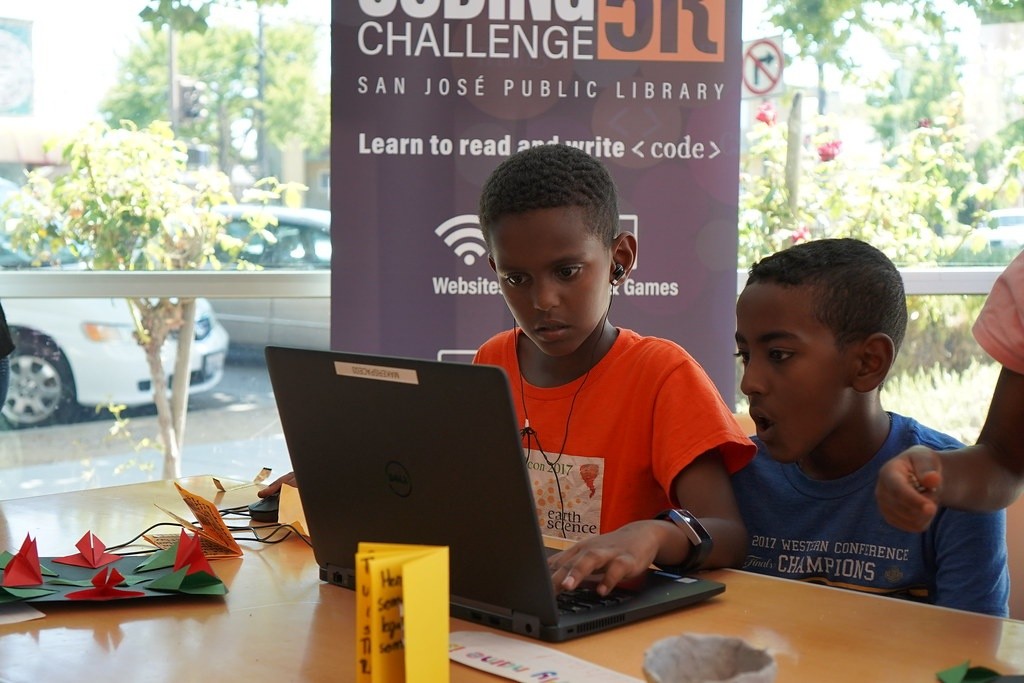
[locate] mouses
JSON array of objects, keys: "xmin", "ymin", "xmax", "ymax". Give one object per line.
[{"xmin": 249, "ymin": 491, "xmax": 280, "ymax": 523}]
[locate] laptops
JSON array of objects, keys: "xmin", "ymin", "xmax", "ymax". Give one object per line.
[{"xmin": 265, "ymin": 345, "xmax": 727, "ymax": 643}]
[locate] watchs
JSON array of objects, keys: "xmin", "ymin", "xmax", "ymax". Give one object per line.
[{"xmin": 652, "ymin": 508, "xmax": 714, "ymax": 575}]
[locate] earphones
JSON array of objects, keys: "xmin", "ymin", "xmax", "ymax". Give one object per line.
[{"xmin": 611, "ymin": 264, "xmax": 625, "ymax": 285}]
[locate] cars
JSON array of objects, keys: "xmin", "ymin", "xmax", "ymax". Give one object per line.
[
  {"xmin": 0, "ymin": 178, "xmax": 228, "ymax": 430},
  {"xmin": 978, "ymin": 208, "xmax": 1024, "ymax": 252},
  {"xmin": 144, "ymin": 205, "xmax": 330, "ymax": 353}
]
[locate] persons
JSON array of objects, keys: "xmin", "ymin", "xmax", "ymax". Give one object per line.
[
  {"xmin": 258, "ymin": 145, "xmax": 757, "ymax": 597},
  {"xmin": 876, "ymin": 249, "xmax": 1024, "ymax": 532},
  {"xmin": 730, "ymin": 238, "xmax": 1010, "ymax": 619}
]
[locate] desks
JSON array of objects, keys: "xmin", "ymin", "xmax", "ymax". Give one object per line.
[{"xmin": 0, "ymin": 474, "xmax": 1024, "ymax": 683}]
